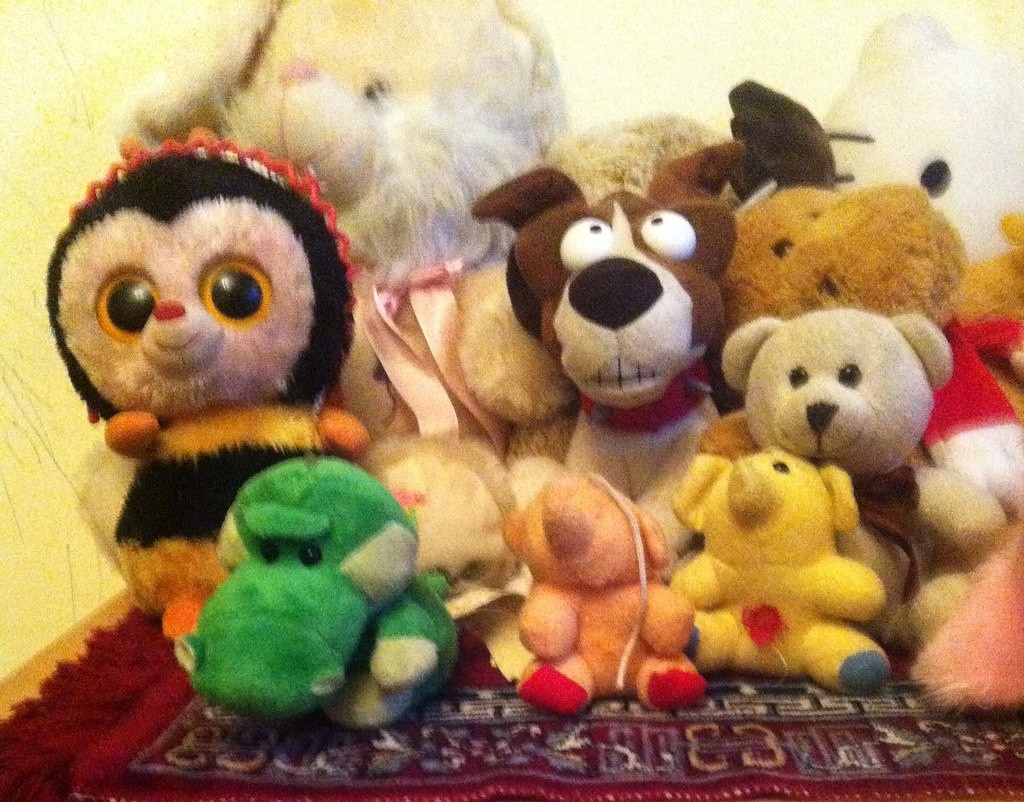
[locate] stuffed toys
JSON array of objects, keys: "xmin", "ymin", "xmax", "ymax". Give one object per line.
[
  {"xmin": 124, "ymin": 2, "xmax": 1023, "ymax": 720},
  {"xmin": 46, "ymin": 132, "xmax": 370, "ymax": 636}
]
